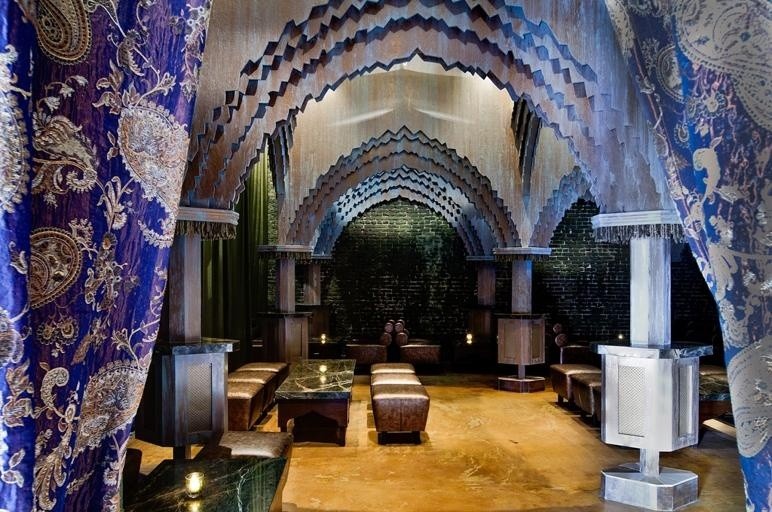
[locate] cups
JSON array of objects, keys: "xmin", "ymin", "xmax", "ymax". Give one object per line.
[{"xmin": 186, "ymin": 471, "xmax": 205, "ymax": 498}]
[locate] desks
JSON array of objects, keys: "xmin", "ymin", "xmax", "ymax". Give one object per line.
[{"xmin": 308, "ymin": 338, "xmax": 343, "ymax": 357}]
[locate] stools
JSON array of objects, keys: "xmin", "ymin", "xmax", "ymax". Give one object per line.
[
  {"xmin": 371, "ymin": 363, "xmax": 430, "ymax": 445},
  {"xmin": 548, "ymin": 362, "xmax": 600, "ymax": 408},
  {"xmin": 121, "ymin": 447, "xmax": 142, "ymax": 502},
  {"xmin": 699, "ymin": 365, "xmax": 728, "ymax": 387},
  {"xmin": 592, "ymin": 385, "xmax": 606, "ymax": 422},
  {"xmin": 193, "ymin": 431, "xmax": 292, "ymax": 463},
  {"xmin": 228, "ymin": 361, "xmax": 289, "ymax": 431},
  {"xmin": 569, "ymin": 372, "xmax": 605, "ymax": 414}
]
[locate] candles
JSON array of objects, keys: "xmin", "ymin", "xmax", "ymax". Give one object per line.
[
  {"xmin": 466, "ymin": 333, "xmax": 474, "ymax": 344},
  {"xmin": 319, "ymin": 364, "xmax": 328, "ymax": 374},
  {"xmin": 321, "ymin": 334, "xmax": 327, "ymax": 341},
  {"xmin": 184, "ymin": 471, "xmax": 206, "ymax": 501}
]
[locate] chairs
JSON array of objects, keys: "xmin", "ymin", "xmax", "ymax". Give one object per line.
[
  {"xmin": 345, "ymin": 319, "xmax": 395, "ymax": 372},
  {"xmin": 394, "ymin": 318, "xmax": 443, "ymax": 370}
]
